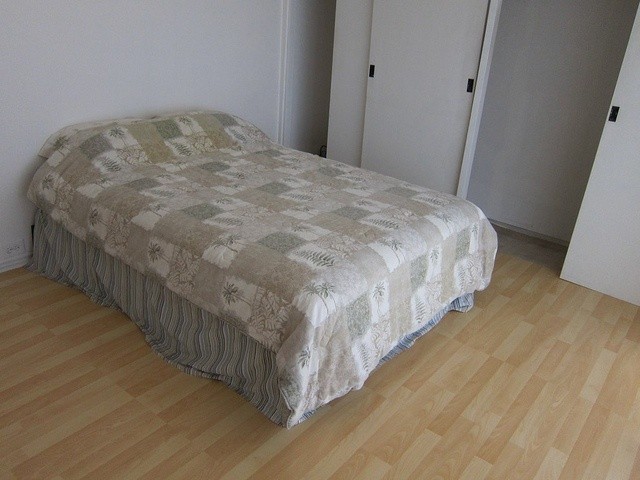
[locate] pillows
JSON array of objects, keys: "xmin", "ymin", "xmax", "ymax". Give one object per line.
[
  {"xmin": 38, "ymin": 117, "xmax": 151, "ymax": 159},
  {"xmin": 23, "ymin": 108, "xmax": 499, "ymax": 432}
]
